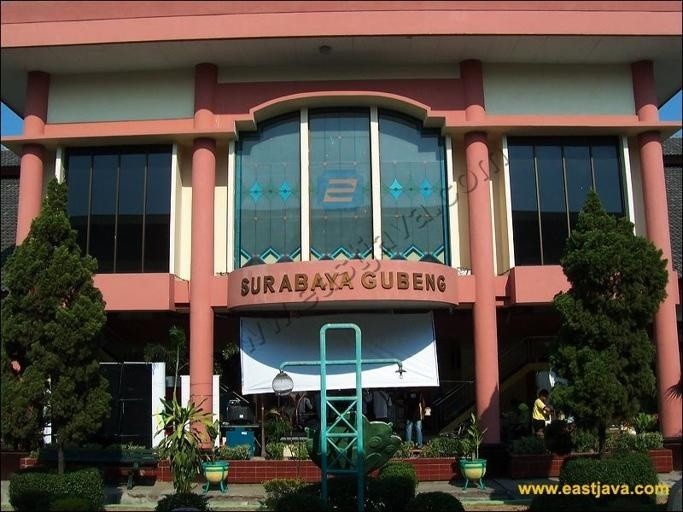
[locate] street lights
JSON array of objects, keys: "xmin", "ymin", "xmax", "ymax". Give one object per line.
[{"xmin": 272, "ymin": 323, "xmax": 406, "ymax": 512}]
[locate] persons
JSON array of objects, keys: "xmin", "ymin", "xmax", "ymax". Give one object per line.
[
  {"xmin": 531, "ymin": 388, "xmax": 550, "ymax": 433},
  {"xmin": 282, "ymin": 388, "xmax": 432, "ymax": 451}
]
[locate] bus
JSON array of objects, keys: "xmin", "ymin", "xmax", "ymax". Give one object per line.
[{"xmin": 280, "ymin": 436, "xmax": 307, "ymax": 444}]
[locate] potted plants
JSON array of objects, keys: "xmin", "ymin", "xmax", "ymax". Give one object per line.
[
  {"xmin": 460, "ymin": 412, "xmax": 488, "ymax": 490},
  {"xmin": 200, "ymin": 420, "xmax": 229, "ymax": 493}
]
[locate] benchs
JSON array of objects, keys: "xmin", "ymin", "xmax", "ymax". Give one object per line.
[{"xmin": 37, "ymin": 447, "xmax": 159, "ymax": 489}]
